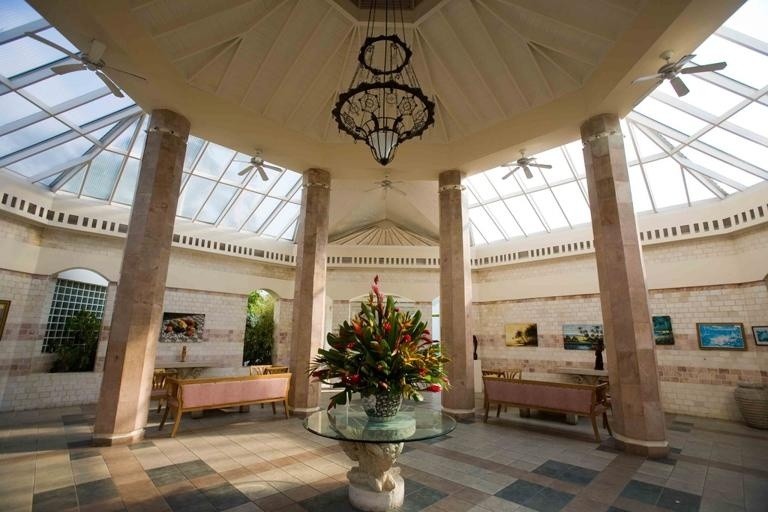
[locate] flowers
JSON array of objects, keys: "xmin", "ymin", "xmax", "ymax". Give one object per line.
[{"xmin": 303, "ymin": 271, "xmax": 453, "ymax": 412}]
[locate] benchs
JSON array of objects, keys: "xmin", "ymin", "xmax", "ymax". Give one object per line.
[
  {"xmin": 483, "ymin": 374, "xmax": 612, "ymax": 444},
  {"xmin": 158, "ymin": 373, "xmax": 293, "ymax": 439}
]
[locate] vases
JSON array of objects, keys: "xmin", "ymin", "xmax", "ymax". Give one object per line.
[{"xmin": 359, "ymin": 386, "xmax": 401, "ymax": 419}]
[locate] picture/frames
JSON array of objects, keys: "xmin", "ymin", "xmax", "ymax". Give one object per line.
[
  {"xmin": 0, "ymin": 300, "xmax": 11, "ymax": 341},
  {"xmin": 696, "ymin": 322, "xmax": 768, "ymax": 351}
]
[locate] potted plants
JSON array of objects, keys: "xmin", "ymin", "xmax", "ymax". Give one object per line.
[{"xmin": 50, "ymin": 306, "xmax": 101, "ymax": 371}]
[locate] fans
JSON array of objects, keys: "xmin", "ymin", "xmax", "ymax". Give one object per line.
[
  {"xmin": 25, "ymin": 26, "xmax": 146, "ymax": 98},
  {"xmin": 630, "ymin": 49, "xmax": 727, "ymax": 98},
  {"xmin": 234, "ymin": 146, "xmax": 282, "ymax": 181},
  {"xmin": 499, "ymin": 148, "xmax": 552, "ymax": 179}
]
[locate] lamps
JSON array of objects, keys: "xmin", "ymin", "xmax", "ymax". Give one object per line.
[{"xmin": 332, "ymin": 0, "xmax": 436, "ymax": 167}]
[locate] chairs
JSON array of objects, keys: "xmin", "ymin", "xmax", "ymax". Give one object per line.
[
  {"xmin": 149, "ymin": 367, "xmax": 168, "ymax": 401},
  {"xmin": 250, "ymin": 365, "xmax": 289, "ymax": 415}
]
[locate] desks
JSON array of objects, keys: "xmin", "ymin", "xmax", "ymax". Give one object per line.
[
  {"xmin": 304, "ymin": 404, "xmax": 458, "ymax": 511},
  {"xmin": 166, "ymin": 362, "xmax": 210, "ymax": 379}
]
